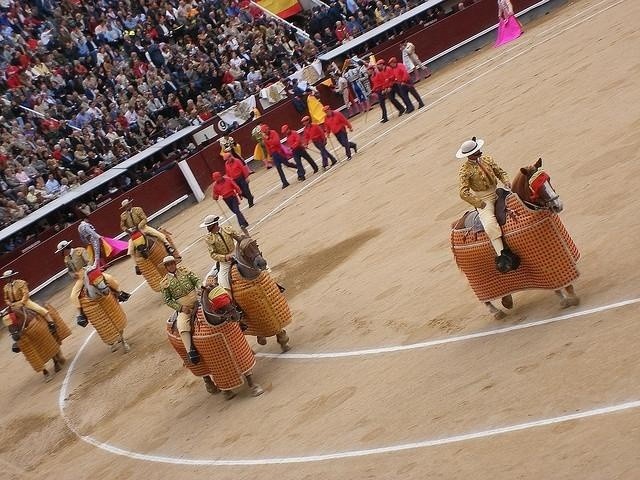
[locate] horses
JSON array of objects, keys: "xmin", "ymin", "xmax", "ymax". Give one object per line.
[
  {"xmin": 202, "ymin": 225, "xmax": 290, "ymax": 353},
  {"xmin": 75, "ymin": 262, "xmax": 132, "ymax": 356},
  {"xmin": 450, "ymin": 157, "xmax": 581, "ymax": 320},
  {"xmin": 123, "ymin": 223, "xmax": 183, "ymax": 292},
  {"xmin": 165, "ymin": 274, "xmax": 266, "ymax": 401},
  {"xmin": 4, "ymin": 300, "xmax": 71, "ymax": 383}
]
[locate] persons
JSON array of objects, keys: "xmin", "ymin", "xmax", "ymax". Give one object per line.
[
  {"xmin": 200, "ymin": 215, "xmax": 287, "ymax": 314},
  {"xmin": 388, "ymin": 57, "xmax": 424, "ymax": 114},
  {"xmin": 292, "ymin": 79, "xmax": 308, "ymax": 114},
  {"xmin": 77, "ymin": 198, "xmax": 115, "ymax": 214},
  {"xmin": 55, "ymin": 240, "xmax": 131, "ymax": 327},
  {"xmin": 78, "ymin": 215, "xmax": 114, "ymax": 272},
  {"xmin": 0, "ymin": 80, "xmax": 197, "ymax": 197},
  {"xmin": 1, "ymin": 199, "xmax": 75, "ymax": 252},
  {"xmin": 159, "ymin": 256, "xmax": 249, "ymax": 364},
  {"xmin": 306, "ymin": 86, "xmax": 326, "ymax": 132},
  {"xmin": 323, "ymin": 105, "xmax": 357, "ymax": 161},
  {"xmin": 0, "ymin": 270, "xmax": 55, "ymax": 352},
  {"xmin": 400, "ymin": 40, "xmax": 432, "ymax": 84},
  {"xmin": 219, "ymin": 132, "xmax": 255, "ymax": 174},
  {"xmin": 1, "ymin": 1, "xmax": 342, "ymax": 80},
  {"xmin": 251, "ymin": 124, "xmax": 272, "ymax": 169},
  {"xmin": 212, "ymin": 172, "xmax": 249, "ymax": 229},
  {"xmin": 280, "ymin": 124, "xmax": 319, "ymax": 181},
  {"xmin": 456, "ymin": 136, "xmax": 520, "ymax": 272},
  {"xmin": 343, "ymin": 0, "xmax": 473, "ymax": 49},
  {"xmin": 118, "ymin": 198, "xmax": 175, "ymax": 276},
  {"xmin": 368, "ymin": 52, "xmax": 378, "ymax": 73},
  {"xmin": 367, "ymin": 64, "xmax": 405, "ymax": 124},
  {"xmin": 260, "ymin": 124, "xmax": 298, "ymax": 189},
  {"xmin": 197, "ymin": 81, "xmax": 258, "ymax": 129},
  {"xmin": 329, "ymin": 72, "xmax": 361, "ymax": 119},
  {"xmin": 356, "ymin": 60, "xmax": 372, "ymax": 94},
  {"xmin": 301, "ymin": 116, "xmax": 337, "ymax": 169},
  {"xmin": 286, "ymin": 86, "xmax": 308, "ymax": 115},
  {"xmin": 375, "ymin": 58, "xmax": 415, "ymax": 117},
  {"xmin": 223, "ymin": 152, "xmax": 255, "ymax": 208},
  {"xmin": 498, "ymin": 0, "xmax": 524, "ymax": 38},
  {"xmin": 342, "ymin": 58, "xmax": 373, "ymax": 113}
]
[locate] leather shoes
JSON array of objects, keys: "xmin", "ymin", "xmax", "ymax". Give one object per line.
[
  {"xmin": 381, "ymin": 119, "xmax": 389, "ymax": 123},
  {"xmin": 354, "ymin": 145, "xmax": 358, "ymax": 154},
  {"xmin": 297, "ymin": 177, "xmax": 306, "ymax": 181},
  {"xmin": 418, "ymin": 103, "xmax": 424, "ymax": 109},
  {"xmin": 282, "ymin": 183, "xmax": 289, "ymax": 188},
  {"xmin": 314, "ymin": 166, "xmax": 318, "ymax": 173},
  {"xmin": 495, "ymin": 256, "xmax": 515, "ymax": 272},
  {"xmin": 323, "ymin": 165, "xmax": 328, "ymax": 169},
  {"xmin": 278, "ymin": 285, "xmax": 285, "ymax": 293},
  {"xmin": 249, "ymin": 202, "xmax": 254, "ymax": 208},
  {"xmin": 12, "ymin": 344, "xmax": 20, "ymax": 352},
  {"xmin": 331, "ymin": 160, "xmax": 337, "ymax": 166},
  {"xmin": 189, "ymin": 352, "xmax": 200, "ymax": 365},
  {"xmin": 48, "ymin": 321, "xmax": 58, "ymax": 331},
  {"xmin": 348, "ymin": 156, "xmax": 351, "ymax": 160},
  {"xmin": 241, "ymin": 222, "xmax": 248, "ymax": 229},
  {"xmin": 77, "ymin": 315, "xmax": 88, "ymax": 327},
  {"xmin": 169, "ymin": 247, "xmax": 175, "ymax": 254},
  {"xmin": 241, "ymin": 323, "xmax": 248, "ymax": 332},
  {"xmin": 405, "ymin": 107, "xmax": 414, "ymax": 113},
  {"xmin": 119, "ymin": 291, "xmax": 131, "ymax": 302},
  {"xmin": 136, "ymin": 267, "xmax": 141, "ymax": 276},
  {"xmin": 399, "ymin": 109, "xmax": 405, "ymax": 116}
]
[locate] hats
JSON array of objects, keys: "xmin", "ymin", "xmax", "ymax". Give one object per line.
[
  {"xmin": 158, "ymin": 256, "xmax": 181, "ymax": 267},
  {"xmin": 212, "ymin": 172, "xmax": 221, "ymax": 179},
  {"xmin": 55, "ymin": 240, "xmax": 73, "ymax": 254},
  {"xmin": 322, "ymin": 105, "xmax": 330, "ymax": 111},
  {"xmin": 301, "ymin": 116, "xmax": 310, "ymax": 122},
  {"xmin": 0, "ymin": 270, "xmax": 19, "ymax": 278},
  {"xmin": 367, "ymin": 65, "xmax": 375, "ymax": 70},
  {"xmin": 261, "ymin": 124, "xmax": 269, "ymax": 131},
  {"xmin": 456, "ymin": 137, "xmax": 484, "ymax": 159},
  {"xmin": 119, "ymin": 199, "xmax": 134, "ymax": 209},
  {"xmin": 223, "ymin": 153, "xmax": 231, "ymax": 160},
  {"xmin": 200, "ymin": 214, "xmax": 223, "ymax": 227},
  {"xmin": 376, "ymin": 59, "xmax": 384, "ymax": 64},
  {"xmin": 388, "ymin": 57, "xmax": 397, "ymax": 64},
  {"xmin": 281, "ymin": 125, "xmax": 288, "ymax": 133}
]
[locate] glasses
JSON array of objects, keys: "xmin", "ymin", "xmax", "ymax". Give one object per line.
[
  {"xmin": 236, "ymin": 87, "xmax": 240, "ymax": 90},
  {"xmin": 145, "ymin": 124, "xmax": 151, "ymax": 126}
]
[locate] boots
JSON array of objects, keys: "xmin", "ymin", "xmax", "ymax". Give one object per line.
[
  {"xmin": 423, "ymin": 68, "xmax": 431, "ymax": 79},
  {"xmin": 96, "ymin": 260, "xmax": 105, "ymax": 272},
  {"xmin": 245, "ymin": 165, "xmax": 255, "ymax": 175},
  {"xmin": 100, "ymin": 258, "xmax": 113, "ymax": 269},
  {"xmin": 365, "ymin": 100, "xmax": 374, "ymax": 111},
  {"xmin": 354, "ymin": 103, "xmax": 360, "ymax": 116},
  {"xmin": 347, "ymin": 108, "xmax": 355, "ymax": 119},
  {"xmin": 414, "ymin": 70, "xmax": 420, "ymax": 83},
  {"xmin": 361, "ymin": 102, "xmax": 366, "ymax": 113},
  {"xmin": 264, "ymin": 159, "xmax": 272, "ymax": 169}
]
